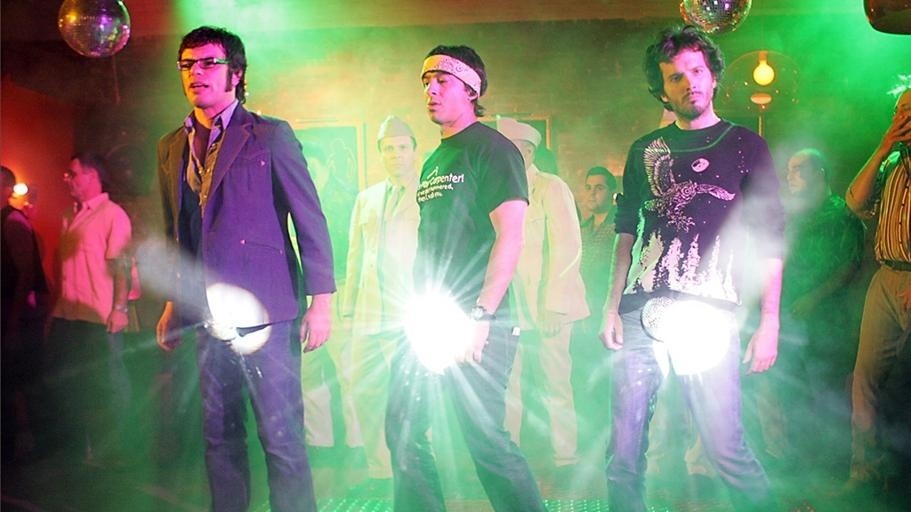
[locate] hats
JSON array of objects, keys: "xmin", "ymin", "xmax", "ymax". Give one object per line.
[
  {"xmin": 376, "ymin": 114, "xmax": 413, "ymax": 139},
  {"xmin": 496, "ymin": 118, "xmax": 541, "ymax": 148}
]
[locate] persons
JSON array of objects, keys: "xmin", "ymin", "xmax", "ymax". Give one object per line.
[
  {"xmin": 340, "ymin": 116, "xmax": 421, "ymax": 490},
  {"xmin": 833, "ymin": 88, "xmax": 911, "ymax": 494},
  {"xmin": 151, "ymin": 26, "xmax": 340, "ymax": 511},
  {"xmin": 0, "ymin": 153, "xmax": 134, "ymax": 512},
  {"xmin": 495, "ymin": 115, "xmax": 617, "ymax": 488},
  {"xmin": 381, "ymin": 40, "xmax": 551, "ymax": 512},
  {"xmin": 773, "ymin": 148, "xmax": 864, "ymax": 482},
  {"xmin": 598, "ymin": 18, "xmax": 791, "ymax": 512}
]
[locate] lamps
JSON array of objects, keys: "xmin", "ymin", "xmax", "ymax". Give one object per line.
[{"xmin": 747, "ymin": 51, "xmax": 780, "ymax": 90}]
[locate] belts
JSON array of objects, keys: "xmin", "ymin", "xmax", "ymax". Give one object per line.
[{"xmin": 883, "ymin": 260, "xmax": 911, "ymax": 271}]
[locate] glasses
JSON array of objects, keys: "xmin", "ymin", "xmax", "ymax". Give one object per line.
[{"xmin": 176, "ymin": 57, "xmax": 230, "ymax": 71}]
